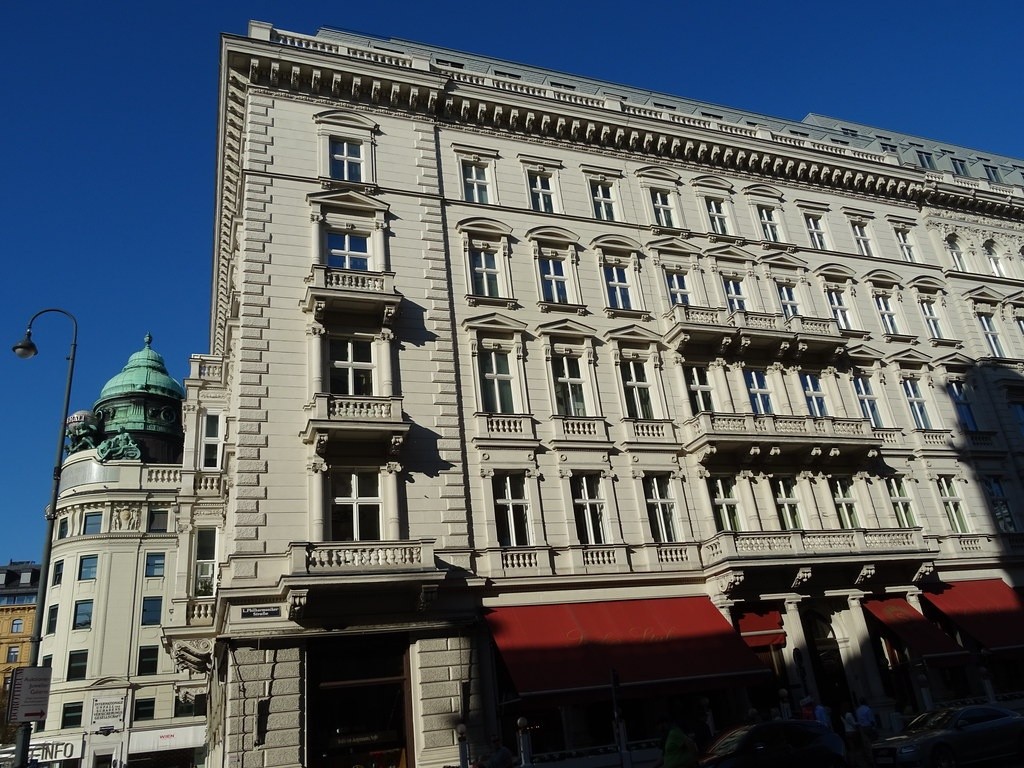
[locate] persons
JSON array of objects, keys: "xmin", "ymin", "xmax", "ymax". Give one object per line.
[
  {"xmin": 838, "ymin": 701, "xmax": 862, "ymax": 768},
  {"xmin": 488, "ymin": 734, "xmax": 513, "ymax": 768},
  {"xmin": 801, "ymin": 696, "xmax": 817, "ymax": 720},
  {"xmin": 694, "ymin": 712, "xmax": 713, "ymax": 754},
  {"xmin": 665, "ymin": 714, "xmax": 699, "ymax": 768},
  {"xmin": 855, "ymin": 697, "xmax": 880, "ymax": 744},
  {"xmin": 812, "ymin": 700, "xmax": 833, "ymax": 731}
]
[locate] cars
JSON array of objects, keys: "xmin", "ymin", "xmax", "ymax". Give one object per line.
[
  {"xmin": 696, "ymin": 718, "xmax": 849, "ymax": 768},
  {"xmin": 874, "ymin": 702, "xmax": 1024, "ymax": 768}
]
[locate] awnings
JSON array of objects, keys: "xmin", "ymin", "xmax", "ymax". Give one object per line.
[
  {"xmin": 861, "ymin": 595, "xmax": 970, "ymax": 658},
  {"xmin": 484, "ymin": 595, "xmax": 786, "ymax": 701},
  {"xmin": 922, "ymin": 579, "xmax": 1024, "ymax": 652}
]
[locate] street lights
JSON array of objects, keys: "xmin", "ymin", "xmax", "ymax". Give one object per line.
[{"xmin": 12, "ymin": 306, "xmax": 80, "ymax": 768}]
